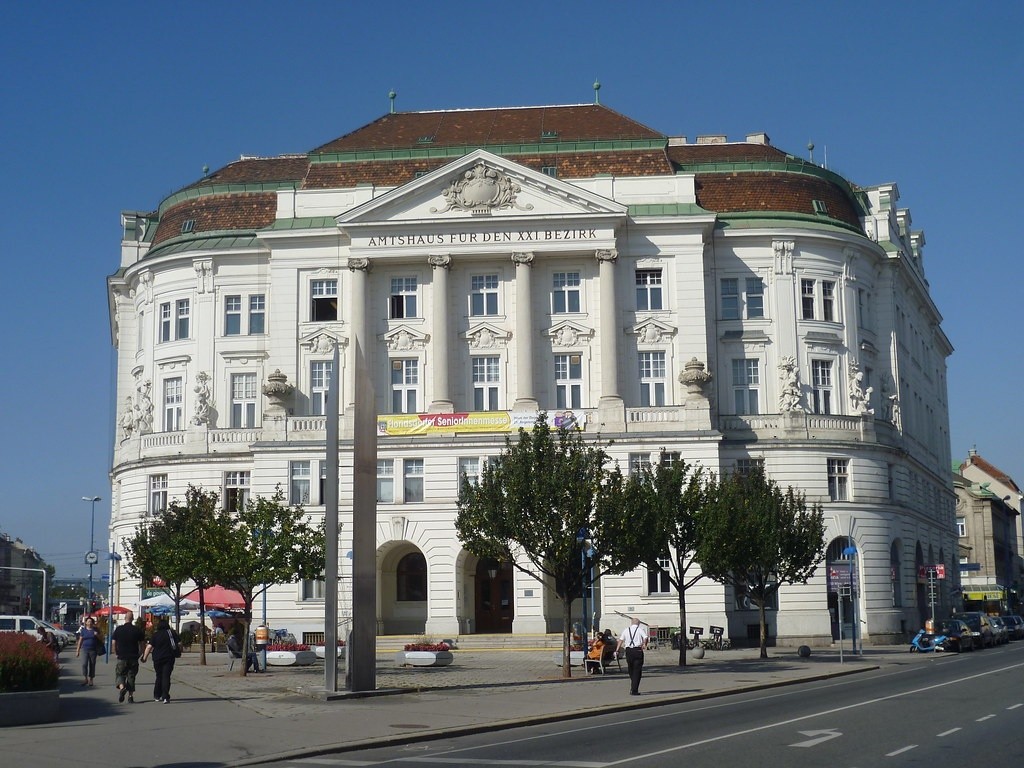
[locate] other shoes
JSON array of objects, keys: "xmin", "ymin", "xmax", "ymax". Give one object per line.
[
  {"xmin": 128, "ymin": 695, "xmax": 133, "ymax": 702},
  {"xmin": 164, "ymin": 698, "xmax": 170, "ymax": 704},
  {"xmin": 89, "ymin": 682, "xmax": 93, "ymax": 686},
  {"xmin": 119, "ymin": 688, "xmax": 126, "ymax": 702},
  {"xmin": 82, "ymin": 679, "xmax": 88, "ymax": 685},
  {"xmin": 155, "ymin": 696, "xmax": 160, "ymax": 701},
  {"xmin": 630, "ymin": 691, "xmax": 640, "ymax": 695}
]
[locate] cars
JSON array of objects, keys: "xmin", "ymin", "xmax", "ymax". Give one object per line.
[
  {"xmin": 921, "ymin": 611, "xmax": 1024, "ymax": 653},
  {"xmin": 0, "ymin": 614, "xmax": 77, "ymax": 653}
]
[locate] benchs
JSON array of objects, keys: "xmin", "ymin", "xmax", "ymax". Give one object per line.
[
  {"xmin": 226, "ymin": 645, "xmax": 252, "ymax": 672},
  {"xmin": 585, "ymin": 644, "xmax": 622, "ymax": 676}
]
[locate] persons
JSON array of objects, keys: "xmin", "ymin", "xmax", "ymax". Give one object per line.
[
  {"xmin": 194, "ymin": 385, "xmax": 211, "ymax": 413},
  {"xmin": 38, "ymin": 627, "xmax": 60, "ymax": 654},
  {"xmin": 783, "ymin": 367, "xmax": 804, "ymax": 411},
  {"xmin": 112, "ymin": 612, "xmax": 145, "ymax": 703},
  {"xmin": 141, "ymin": 620, "xmax": 182, "ymax": 703},
  {"xmin": 228, "ymin": 628, "xmax": 263, "ymax": 673},
  {"xmin": 852, "ymin": 371, "xmax": 874, "ymax": 415},
  {"xmin": 76, "ymin": 618, "xmax": 103, "ymax": 686},
  {"xmin": 212, "ymin": 626, "xmax": 223, "ymax": 634},
  {"xmin": 219, "ymin": 623, "xmax": 224, "ymax": 632},
  {"xmin": 580, "ymin": 618, "xmax": 648, "ymax": 695},
  {"xmin": 118, "ymin": 397, "xmax": 153, "ymax": 439}
]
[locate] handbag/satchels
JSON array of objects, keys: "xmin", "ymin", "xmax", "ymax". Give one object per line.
[
  {"xmin": 168, "ymin": 629, "xmax": 181, "ymax": 658},
  {"xmin": 95, "ymin": 636, "xmax": 105, "ymax": 656}
]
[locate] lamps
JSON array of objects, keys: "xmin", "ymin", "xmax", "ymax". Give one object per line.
[
  {"xmin": 1002, "ymin": 495, "xmax": 1010, "ymax": 503},
  {"xmin": 487, "ymin": 559, "xmax": 499, "ymax": 584}
]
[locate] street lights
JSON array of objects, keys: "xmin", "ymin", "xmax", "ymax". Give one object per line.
[
  {"xmin": 251, "ymin": 527, "xmax": 274, "ymax": 670},
  {"xmin": 584, "ymin": 536, "xmax": 598, "ymax": 642},
  {"xmin": 80, "ymin": 495, "xmax": 103, "ymax": 617},
  {"xmin": 102, "ymin": 541, "xmax": 123, "ymax": 654},
  {"xmin": 841, "ymin": 535, "xmax": 857, "ymax": 654}
]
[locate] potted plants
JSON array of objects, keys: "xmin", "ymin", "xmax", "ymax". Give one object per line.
[
  {"xmin": 212, "ymin": 627, "xmax": 235, "ymax": 652},
  {"xmin": 191, "ymin": 632, "xmax": 212, "ymax": 653},
  {"xmin": 403, "ymin": 643, "xmax": 453, "ymax": 665},
  {"xmin": 316, "ymin": 640, "xmax": 347, "ymax": 658},
  {"xmin": 179, "ymin": 631, "xmax": 194, "ymax": 653},
  {"xmin": 266, "ymin": 644, "xmax": 316, "ymax": 665}
]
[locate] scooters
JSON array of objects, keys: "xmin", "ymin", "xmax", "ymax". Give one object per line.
[{"xmin": 909, "ymin": 626, "xmax": 951, "ymax": 653}]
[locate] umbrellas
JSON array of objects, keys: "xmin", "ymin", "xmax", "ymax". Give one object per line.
[
  {"xmin": 95, "ymin": 607, "xmax": 132, "ymax": 615},
  {"xmin": 136, "ymin": 595, "xmax": 200, "ymax": 607},
  {"xmin": 197, "ymin": 608, "xmax": 232, "ymax": 627},
  {"xmin": 144, "ymin": 605, "xmax": 189, "ymax": 616}
]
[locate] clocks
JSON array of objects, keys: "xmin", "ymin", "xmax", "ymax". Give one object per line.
[{"xmin": 84, "ymin": 551, "xmax": 98, "ymax": 564}]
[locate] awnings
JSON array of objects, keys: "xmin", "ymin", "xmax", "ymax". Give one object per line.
[
  {"xmin": 1005, "ymin": 587, "xmax": 1019, "ymax": 600},
  {"xmin": 962, "ymin": 585, "xmax": 1007, "ymax": 600},
  {"xmin": 184, "ymin": 587, "xmax": 245, "ymax": 608}
]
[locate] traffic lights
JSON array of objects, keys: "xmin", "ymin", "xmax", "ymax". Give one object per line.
[
  {"xmin": 89, "ymin": 600, "xmax": 96, "ymax": 613},
  {"xmin": 25, "ymin": 598, "xmax": 31, "ymax": 612}
]
